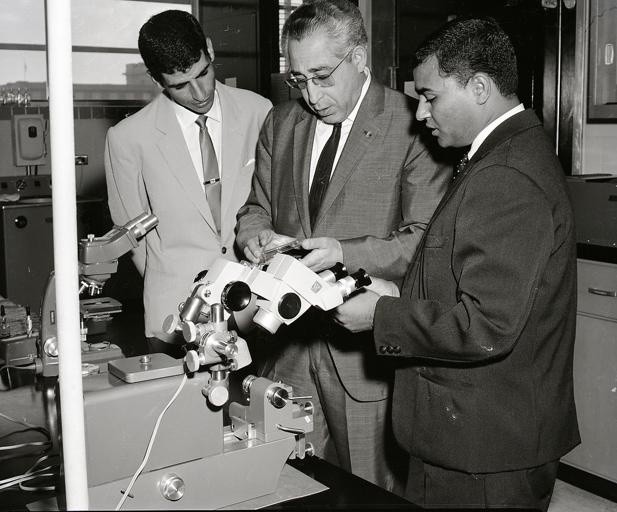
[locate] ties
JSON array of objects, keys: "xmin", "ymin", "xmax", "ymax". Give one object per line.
[
  {"xmin": 309, "ymin": 122, "xmax": 343, "ymax": 234},
  {"xmin": 195, "ymin": 116, "xmax": 222, "ymax": 235},
  {"xmin": 446, "ymin": 152, "xmax": 470, "ymax": 190}
]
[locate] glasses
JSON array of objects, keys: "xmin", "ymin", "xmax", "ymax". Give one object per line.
[{"xmin": 284, "ymin": 49, "xmax": 354, "ymax": 91}]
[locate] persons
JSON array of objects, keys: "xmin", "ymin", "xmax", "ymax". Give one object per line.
[
  {"xmin": 329, "ymin": 14, "xmax": 582, "ymax": 512},
  {"xmin": 104, "ymin": 9, "xmax": 274, "ymax": 360},
  {"xmin": 230, "ymin": 2, "xmax": 454, "ymax": 498}
]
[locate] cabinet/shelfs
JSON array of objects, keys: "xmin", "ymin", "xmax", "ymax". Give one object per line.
[{"xmin": 553, "ymin": 249, "xmax": 617, "ymax": 483}]
